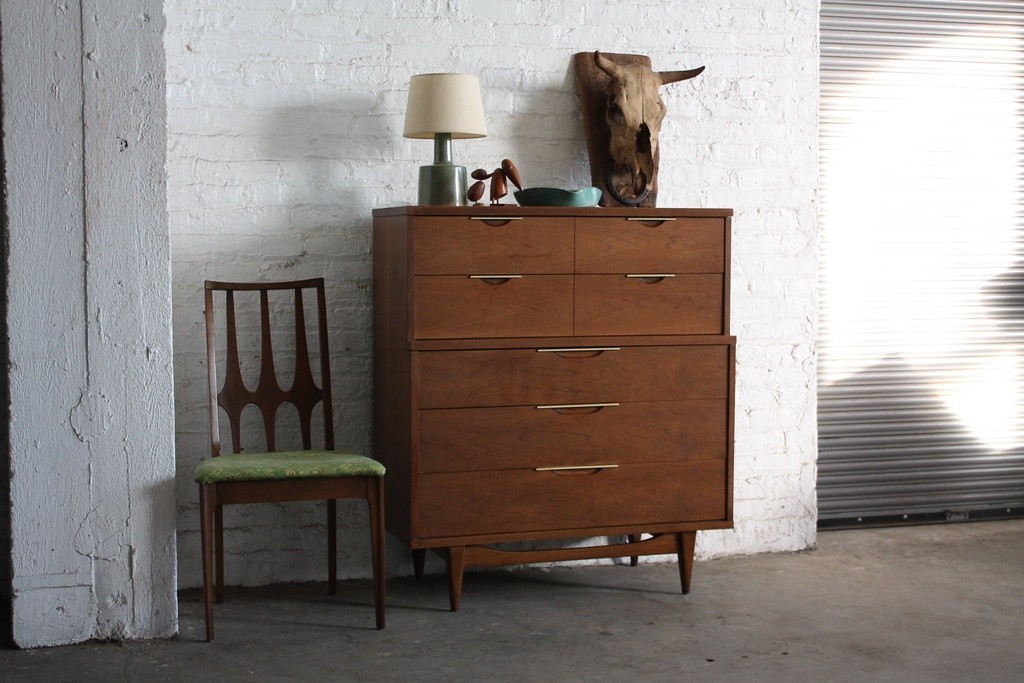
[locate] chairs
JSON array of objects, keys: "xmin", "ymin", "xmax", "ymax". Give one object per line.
[{"xmin": 195, "ymin": 277, "xmax": 386, "ymax": 642}]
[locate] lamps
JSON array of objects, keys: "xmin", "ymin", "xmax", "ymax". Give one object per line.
[{"xmin": 403, "ymin": 73, "xmax": 488, "ymax": 206}]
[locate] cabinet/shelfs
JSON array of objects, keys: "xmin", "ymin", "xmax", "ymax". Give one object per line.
[{"xmin": 369, "ymin": 206, "xmax": 736, "ymax": 611}]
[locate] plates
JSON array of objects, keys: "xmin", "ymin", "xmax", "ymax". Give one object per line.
[{"xmin": 513, "ymin": 187, "xmax": 603, "ymax": 207}]
